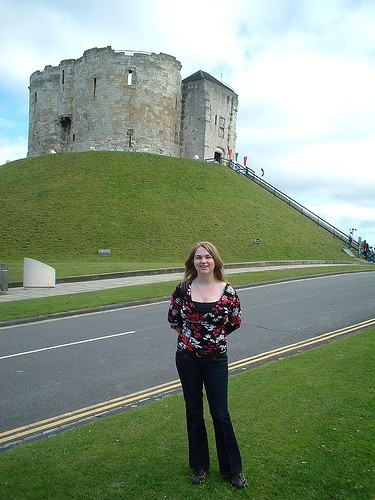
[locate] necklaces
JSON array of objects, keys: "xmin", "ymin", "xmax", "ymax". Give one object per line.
[{"xmin": 194, "ymin": 279, "xmax": 216, "ymax": 302}]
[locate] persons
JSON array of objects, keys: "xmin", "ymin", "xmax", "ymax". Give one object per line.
[
  {"xmin": 167, "ymin": 241, "xmax": 248, "ymax": 489},
  {"xmin": 348, "ymin": 232, "xmax": 352, "ymax": 249},
  {"xmin": 362, "ymin": 240, "xmax": 375, "ymax": 263}
]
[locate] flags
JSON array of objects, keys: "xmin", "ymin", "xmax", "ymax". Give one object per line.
[{"xmin": 228, "ymin": 150, "xmax": 248, "ymax": 166}]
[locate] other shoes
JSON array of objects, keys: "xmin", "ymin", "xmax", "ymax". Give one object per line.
[
  {"xmin": 231, "ymin": 472, "xmax": 250, "ymax": 490},
  {"xmin": 190, "ymin": 468, "xmax": 207, "ymax": 484}
]
